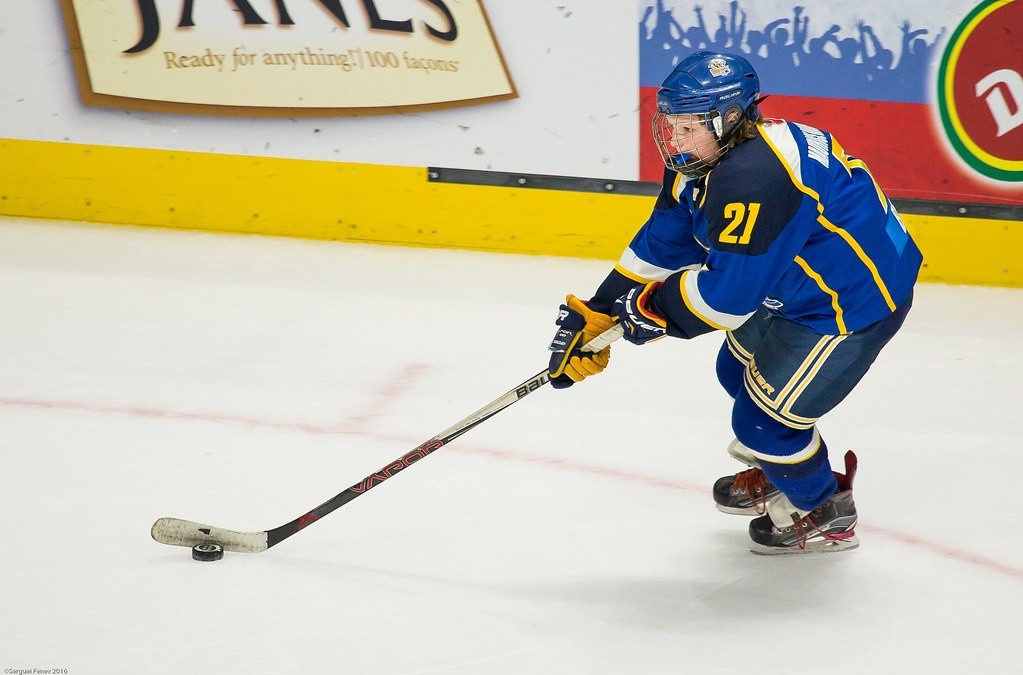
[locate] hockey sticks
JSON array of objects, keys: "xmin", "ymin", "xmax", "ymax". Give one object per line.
[{"xmin": 151, "ymin": 322, "xmax": 624, "ymax": 553}]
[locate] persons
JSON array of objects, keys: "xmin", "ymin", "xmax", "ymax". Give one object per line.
[{"xmin": 550, "ymin": 51, "xmax": 925, "ymax": 548}]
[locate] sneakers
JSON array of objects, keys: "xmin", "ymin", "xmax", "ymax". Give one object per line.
[{"xmin": 713, "ymin": 439, "xmax": 862, "ymax": 556}]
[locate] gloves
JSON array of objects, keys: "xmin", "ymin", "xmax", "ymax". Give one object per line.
[
  {"xmin": 545, "ymin": 293, "xmax": 615, "ymax": 387},
  {"xmin": 612, "ymin": 280, "xmax": 668, "ymax": 346}
]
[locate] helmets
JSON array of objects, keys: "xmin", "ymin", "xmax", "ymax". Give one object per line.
[{"xmin": 650, "ymin": 51, "xmax": 759, "ymax": 178}]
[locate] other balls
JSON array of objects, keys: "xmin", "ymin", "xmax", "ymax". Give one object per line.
[{"xmin": 192, "ymin": 543, "xmax": 224, "ymax": 562}]
